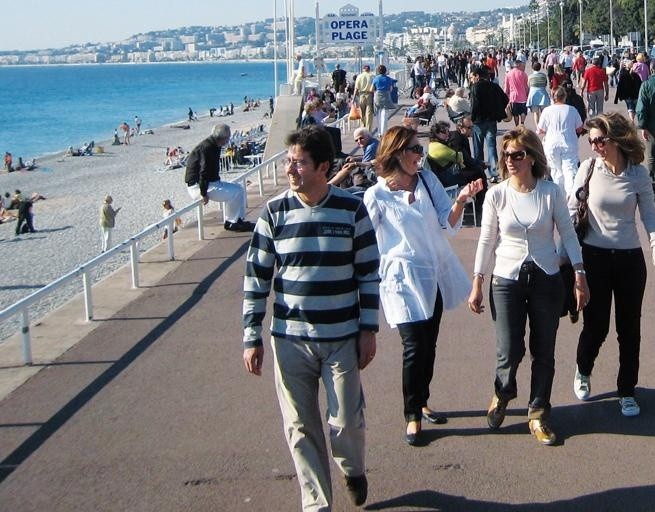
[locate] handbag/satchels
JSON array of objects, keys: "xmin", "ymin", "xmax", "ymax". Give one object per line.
[
  {"xmin": 569, "ymin": 188, "xmax": 590, "ymax": 242},
  {"xmin": 392, "ymin": 81, "xmax": 399, "ymax": 103},
  {"xmin": 504, "ymin": 102, "xmax": 512, "ymax": 122},
  {"xmin": 447, "ymin": 163, "xmax": 460, "ymax": 176}
]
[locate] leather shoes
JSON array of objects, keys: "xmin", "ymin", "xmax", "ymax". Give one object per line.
[
  {"xmin": 423, "ymin": 413, "xmax": 441, "ymax": 423},
  {"xmin": 406, "ymin": 421, "xmax": 422, "ymax": 446},
  {"xmin": 344, "ymin": 473, "xmax": 369, "ymax": 507}
]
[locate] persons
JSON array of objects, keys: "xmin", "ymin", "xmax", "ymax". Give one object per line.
[
  {"xmin": 557, "ymin": 112, "xmax": 654, "ymax": 416},
  {"xmin": 163, "ymin": 44, "xmax": 655, "ymax": 255},
  {"xmin": 241, "ymin": 126, "xmax": 382, "ymax": 511},
  {"xmin": 114, "ymin": 116, "xmax": 155, "ymax": 144},
  {"xmin": 100, "ymin": 195, "xmax": 121, "ymax": 254},
  {"xmin": 362, "ymin": 125, "xmax": 485, "ymax": 446},
  {"xmin": 64, "ymin": 140, "xmax": 95, "ymax": 157},
  {"xmin": 1, "ymin": 190, "xmax": 46, "ymax": 237},
  {"xmin": 467, "ymin": 128, "xmax": 591, "ymax": 446},
  {"xmin": 4, "ymin": 152, "xmax": 37, "ymax": 172},
  {"xmin": 162, "ymin": 200, "xmax": 178, "ymax": 239}
]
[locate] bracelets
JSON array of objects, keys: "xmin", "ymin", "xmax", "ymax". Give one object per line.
[
  {"xmin": 472, "ymin": 273, "xmax": 484, "ymax": 282},
  {"xmin": 574, "ymin": 269, "xmax": 586, "ymax": 275}
]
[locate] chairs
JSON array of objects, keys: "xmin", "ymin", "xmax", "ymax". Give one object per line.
[{"xmin": 421, "ymin": 154, "xmax": 477, "ymax": 226}]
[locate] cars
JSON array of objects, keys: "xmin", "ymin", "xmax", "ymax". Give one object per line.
[{"xmin": 533, "ymin": 43, "xmax": 655, "ymax": 62}]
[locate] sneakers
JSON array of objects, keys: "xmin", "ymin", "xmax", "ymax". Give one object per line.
[
  {"xmin": 619, "ymin": 396, "xmax": 641, "ymax": 416},
  {"xmin": 224, "ymin": 222, "xmax": 237, "ymax": 229},
  {"xmin": 488, "ymin": 395, "xmax": 510, "ymax": 431},
  {"xmin": 490, "ymin": 177, "xmax": 497, "ymax": 183},
  {"xmin": 574, "ymin": 365, "xmax": 591, "ymax": 402},
  {"xmin": 529, "ymin": 419, "xmax": 557, "ymax": 445}
]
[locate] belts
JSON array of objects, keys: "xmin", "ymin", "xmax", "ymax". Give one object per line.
[{"xmin": 521, "ymin": 262, "xmax": 542, "ymax": 275}]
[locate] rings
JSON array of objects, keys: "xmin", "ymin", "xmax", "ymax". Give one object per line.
[{"xmin": 369, "ymin": 353, "xmax": 374, "ymax": 360}]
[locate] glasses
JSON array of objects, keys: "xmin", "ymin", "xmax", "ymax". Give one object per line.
[
  {"xmin": 355, "ymin": 136, "xmax": 364, "ymax": 142},
  {"xmin": 406, "ymin": 144, "xmax": 424, "ymax": 155},
  {"xmin": 282, "ymin": 158, "xmax": 314, "ymax": 169},
  {"xmin": 465, "ymin": 126, "xmax": 475, "ymax": 130},
  {"xmin": 503, "ymin": 151, "xmax": 531, "ymax": 163},
  {"xmin": 588, "ymin": 137, "xmax": 610, "ymax": 149}
]
